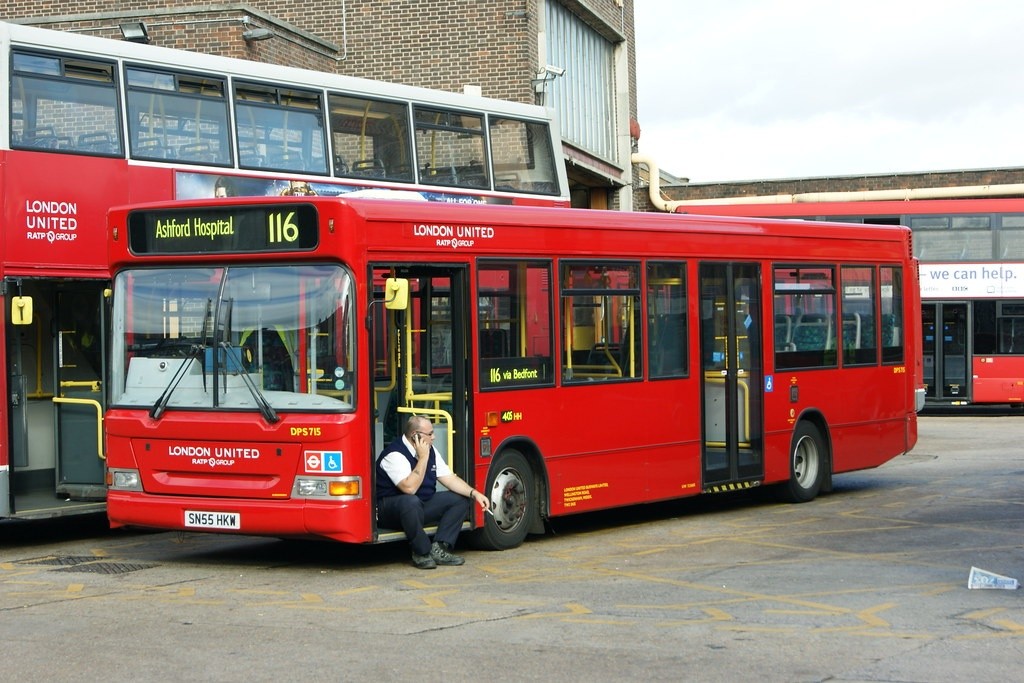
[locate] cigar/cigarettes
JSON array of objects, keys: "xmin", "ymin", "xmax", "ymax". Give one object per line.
[{"xmin": 484, "ymin": 507, "xmax": 493, "ymax": 515}]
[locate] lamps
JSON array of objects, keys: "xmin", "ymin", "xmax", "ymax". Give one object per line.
[
  {"xmin": 242, "ymin": 27, "xmax": 274, "ymax": 42},
  {"xmin": 119, "ymin": 21, "xmax": 152, "ymax": 44}
]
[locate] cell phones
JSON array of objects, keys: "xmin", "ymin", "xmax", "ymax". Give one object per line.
[{"xmin": 412, "ymin": 434, "xmax": 422, "ymax": 444}]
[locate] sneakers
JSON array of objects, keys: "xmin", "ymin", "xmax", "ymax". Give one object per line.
[{"xmin": 432, "ymin": 545, "xmax": 464, "ymax": 566}]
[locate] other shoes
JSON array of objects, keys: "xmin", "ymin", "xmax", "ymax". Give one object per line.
[{"xmin": 411, "ymin": 551, "xmax": 437, "ymax": 569}]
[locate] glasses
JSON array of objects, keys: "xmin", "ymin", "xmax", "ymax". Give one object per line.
[{"xmin": 417, "ymin": 430, "xmax": 434, "ymax": 436}]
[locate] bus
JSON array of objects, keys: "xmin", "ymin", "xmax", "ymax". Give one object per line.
[
  {"xmin": 0, "ymin": 22, "xmax": 569, "ymax": 526},
  {"xmin": 106, "ymin": 197, "xmax": 918, "ymax": 550},
  {"xmin": 674, "ymin": 201, "xmax": 1023, "ymax": 405}
]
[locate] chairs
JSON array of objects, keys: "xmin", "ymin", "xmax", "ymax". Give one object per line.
[
  {"xmin": 387, "ymin": 164, "xmax": 412, "ymax": 180},
  {"xmin": 312, "ymin": 156, "xmax": 346, "ymax": 175},
  {"xmin": 77, "ymin": 132, "xmax": 114, "ymax": 154},
  {"xmin": 830, "ymin": 312, "xmax": 861, "ymax": 349},
  {"xmin": 494, "ymin": 173, "xmax": 521, "ymax": 189},
  {"xmin": 861, "ymin": 314, "xmax": 895, "ymax": 348},
  {"xmin": 239, "ymin": 324, "xmax": 295, "ymax": 391},
  {"xmin": 424, "ymin": 166, "xmax": 457, "ymax": 184},
  {"xmin": 586, "ymin": 343, "xmax": 623, "ymax": 374},
  {"xmin": 178, "ymin": 142, "xmax": 218, "ymax": 162},
  {"xmin": 464, "ymin": 173, "xmax": 496, "ymax": 187},
  {"xmin": 480, "ymin": 328, "xmax": 508, "ymax": 372},
  {"xmin": 22, "ymin": 126, "xmax": 59, "ymax": 150},
  {"xmin": 352, "ymin": 159, "xmax": 385, "ymax": 178},
  {"xmin": 240, "ymin": 146, "xmax": 262, "ymax": 166},
  {"xmin": 271, "ymin": 151, "xmax": 306, "ymax": 171},
  {"xmin": 133, "ymin": 137, "xmax": 166, "ymax": 158},
  {"xmin": 775, "ymin": 314, "xmax": 830, "ymax": 350}
]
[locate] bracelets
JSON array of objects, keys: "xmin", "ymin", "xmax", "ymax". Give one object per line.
[{"xmin": 470, "ymin": 489, "xmax": 475, "ymax": 498}]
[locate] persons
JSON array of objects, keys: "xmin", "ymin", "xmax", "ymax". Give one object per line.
[{"xmin": 376, "ymin": 416, "xmax": 490, "ymax": 569}]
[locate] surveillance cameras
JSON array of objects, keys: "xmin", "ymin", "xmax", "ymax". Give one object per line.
[{"xmin": 545, "ymin": 65, "xmax": 566, "ymax": 77}]
